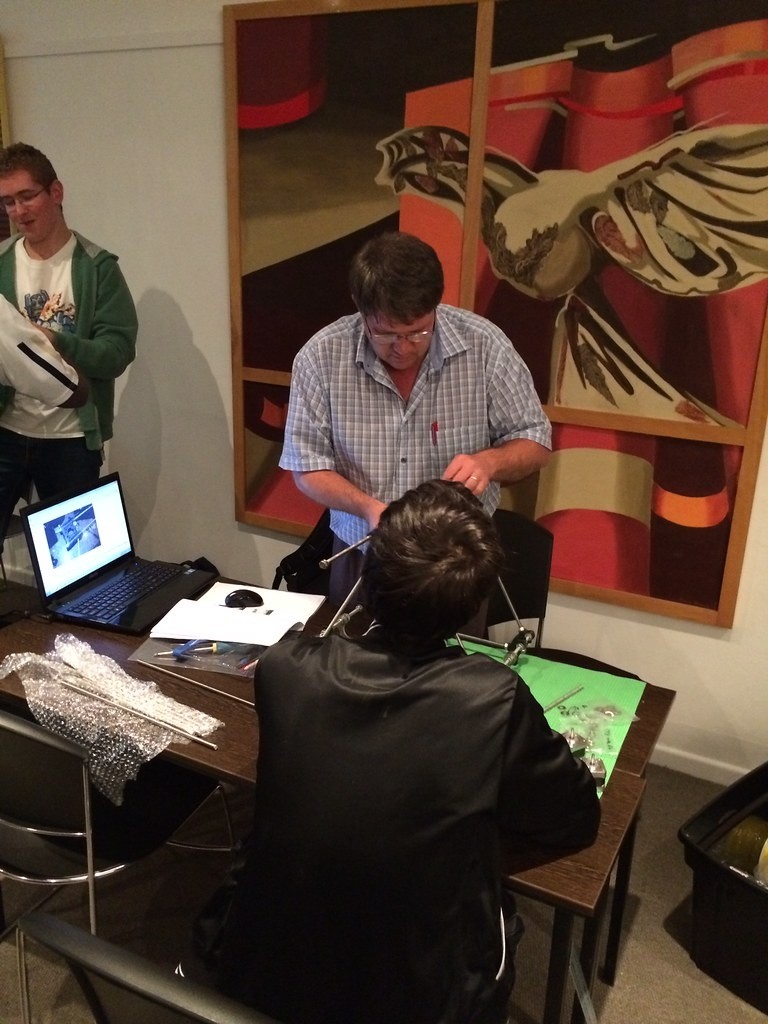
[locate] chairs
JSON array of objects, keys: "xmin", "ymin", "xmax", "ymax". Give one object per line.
[
  {"xmin": 16, "ymin": 909, "xmax": 284, "ymax": 1024},
  {"xmin": 485, "ymin": 505, "xmax": 554, "ymax": 650},
  {"xmin": 0, "ymin": 710, "xmax": 234, "ymax": 937}
]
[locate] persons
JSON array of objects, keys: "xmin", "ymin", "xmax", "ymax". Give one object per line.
[
  {"xmin": 279, "ymin": 233, "xmax": 553, "ymax": 639},
  {"xmin": 0, "ymin": 142, "xmax": 139, "ymax": 551},
  {"xmin": 206, "ymin": 480, "xmax": 601, "ymax": 1024}
]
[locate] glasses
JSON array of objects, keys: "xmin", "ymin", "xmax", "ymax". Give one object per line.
[
  {"xmin": 366, "ymin": 319, "xmax": 435, "ymax": 344},
  {"xmin": 4, "ymin": 186, "xmax": 47, "ymax": 209}
]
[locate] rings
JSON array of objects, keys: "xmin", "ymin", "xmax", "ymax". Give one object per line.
[{"xmin": 471, "ymin": 476, "xmax": 477, "ymax": 480}]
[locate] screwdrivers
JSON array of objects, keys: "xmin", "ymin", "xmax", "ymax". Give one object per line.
[{"xmin": 152, "ymin": 642, "xmax": 233, "ymax": 658}]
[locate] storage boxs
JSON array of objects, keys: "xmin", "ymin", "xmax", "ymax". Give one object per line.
[{"xmin": 677, "ymin": 761, "xmax": 768, "ymax": 1015}]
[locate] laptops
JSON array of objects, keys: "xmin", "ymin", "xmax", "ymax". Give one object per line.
[{"xmin": 19, "ymin": 472, "xmax": 217, "ymax": 637}]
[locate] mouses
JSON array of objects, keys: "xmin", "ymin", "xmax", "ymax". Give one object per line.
[{"xmin": 225, "ymin": 590, "xmax": 263, "ymax": 608}]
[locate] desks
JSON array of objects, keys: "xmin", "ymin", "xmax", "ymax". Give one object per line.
[
  {"xmin": 30, "ymin": 560, "xmax": 676, "ymax": 987},
  {"xmin": 0, "ymin": 616, "xmax": 646, "ymax": 1024}
]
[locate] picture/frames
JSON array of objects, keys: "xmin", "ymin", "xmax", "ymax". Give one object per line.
[{"xmin": 221, "ymin": 0, "xmax": 768, "ymax": 629}]
